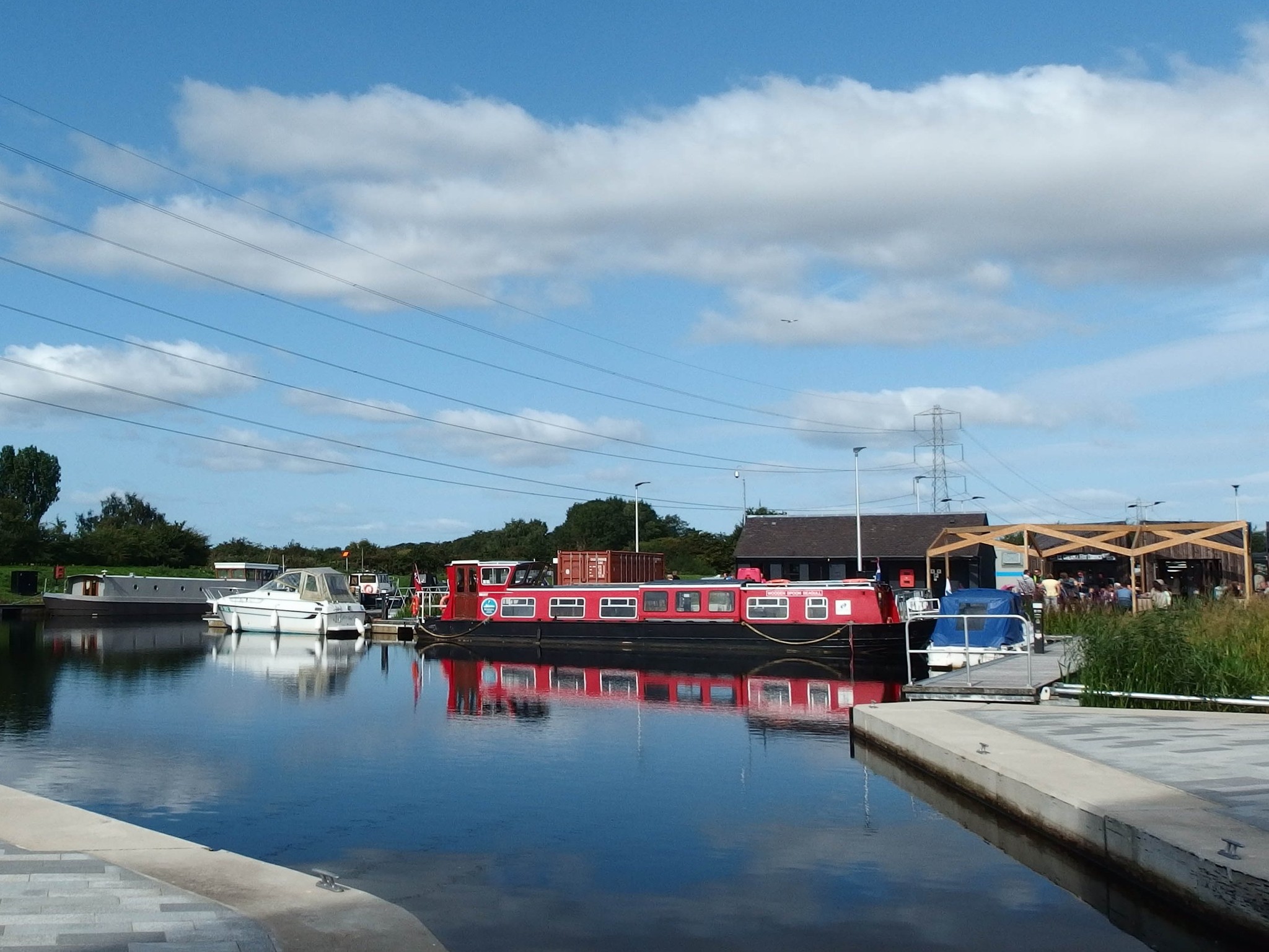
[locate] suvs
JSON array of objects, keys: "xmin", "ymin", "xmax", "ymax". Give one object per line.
[{"xmin": 347, "ymin": 570, "xmax": 397, "ymax": 597}]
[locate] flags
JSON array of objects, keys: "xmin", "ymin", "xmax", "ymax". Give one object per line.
[{"xmin": 414, "ymin": 567, "xmax": 424, "ymax": 596}]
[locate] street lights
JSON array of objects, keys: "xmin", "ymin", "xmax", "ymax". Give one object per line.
[
  {"xmin": 735, "ymin": 464, "xmax": 747, "ymax": 527},
  {"xmin": 635, "ymin": 481, "xmax": 651, "ymax": 553},
  {"xmin": 915, "ymin": 476, "xmax": 927, "ymax": 513},
  {"xmin": 1128, "ymin": 500, "xmax": 1167, "ymax": 521},
  {"xmin": 941, "ymin": 496, "xmax": 985, "ymax": 513},
  {"xmin": 1232, "ymin": 485, "xmax": 1241, "ymax": 521},
  {"xmin": 853, "ymin": 446, "xmax": 867, "ymax": 572}
]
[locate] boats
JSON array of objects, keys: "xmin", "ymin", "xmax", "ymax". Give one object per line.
[
  {"xmin": 41, "ymin": 620, "xmax": 218, "ymax": 659},
  {"xmin": 201, "ymin": 564, "xmax": 435, "ymax": 639},
  {"xmin": 413, "ymin": 558, "xmax": 942, "ymax": 681},
  {"xmin": 924, "ymin": 584, "xmax": 1037, "ymax": 667},
  {"xmin": 437, "ymin": 657, "xmax": 914, "ymax": 747},
  {"xmin": 202, "ymin": 626, "xmax": 372, "ymax": 697},
  {"xmin": 43, "ymin": 561, "xmax": 280, "ymax": 616}
]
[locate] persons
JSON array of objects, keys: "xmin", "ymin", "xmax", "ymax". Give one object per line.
[
  {"xmin": 1010, "ymin": 557, "xmax": 1268, "ymax": 618},
  {"xmin": 672, "ymin": 571, "xmax": 682, "ymax": 610},
  {"xmin": 722, "ymin": 573, "xmax": 737, "ymax": 613}
]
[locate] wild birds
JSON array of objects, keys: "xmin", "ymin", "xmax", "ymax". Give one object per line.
[{"xmin": 781, "ymin": 318, "xmax": 799, "ymax": 323}]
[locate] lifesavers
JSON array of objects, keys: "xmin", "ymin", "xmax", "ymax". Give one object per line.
[
  {"xmin": 440, "ymin": 596, "xmax": 450, "ymax": 610},
  {"xmin": 766, "ymin": 579, "xmax": 789, "ymax": 586},
  {"xmin": 412, "ymin": 596, "xmax": 418, "ymax": 615},
  {"xmin": 844, "ymin": 579, "xmax": 867, "ymax": 582}
]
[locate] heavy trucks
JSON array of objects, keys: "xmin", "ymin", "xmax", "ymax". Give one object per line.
[{"xmin": 556, "ymin": 551, "xmax": 666, "ymax": 584}]
